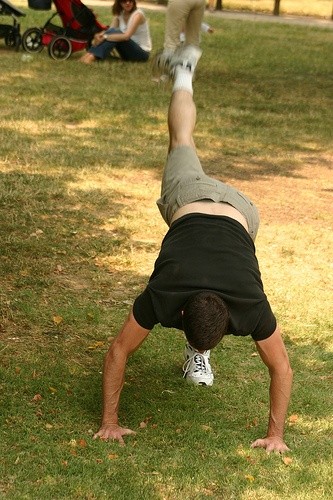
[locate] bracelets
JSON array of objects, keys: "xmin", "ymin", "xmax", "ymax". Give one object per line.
[{"xmin": 103, "ymin": 35, "xmax": 108, "ymax": 40}]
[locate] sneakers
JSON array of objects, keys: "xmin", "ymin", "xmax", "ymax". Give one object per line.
[
  {"xmin": 152, "ymin": 43, "xmax": 204, "ymax": 73},
  {"xmin": 183, "ymin": 341, "xmax": 213, "ymax": 386}
]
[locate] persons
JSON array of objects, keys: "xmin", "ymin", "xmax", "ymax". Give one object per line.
[
  {"xmin": 180, "ymin": 19, "xmax": 214, "ymax": 44},
  {"xmin": 91, "ymin": 41, "xmax": 293, "ymax": 457},
  {"xmin": 163, "ymin": 0, "xmax": 205, "ymax": 51},
  {"xmin": 79, "ymin": 0, "xmax": 152, "ymax": 64}
]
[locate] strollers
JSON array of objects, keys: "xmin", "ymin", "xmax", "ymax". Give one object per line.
[{"xmin": 0, "ymin": 0, "xmax": 110, "ymax": 61}]
